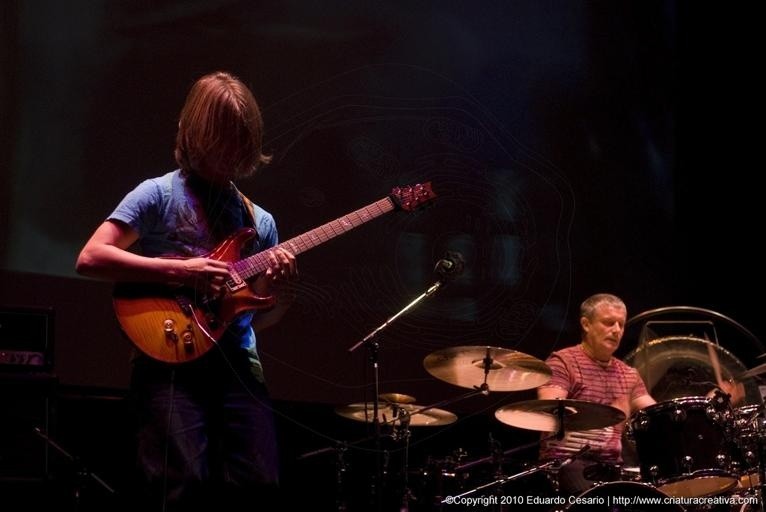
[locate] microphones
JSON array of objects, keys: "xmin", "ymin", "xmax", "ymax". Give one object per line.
[{"xmin": 439, "ymin": 252, "xmax": 466, "ymax": 282}]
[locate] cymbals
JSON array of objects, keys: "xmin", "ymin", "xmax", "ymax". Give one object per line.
[
  {"xmin": 493, "ymin": 400, "xmax": 625, "ymax": 432},
  {"xmin": 337, "ymin": 401, "xmax": 457, "ymax": 426},
  {"xmin": 379, "ymin": 392, "xmax": 415, "ymax": 404},
  {"xmin": 422, "ymin": 345, "xmax": 552, "ymax": 392}
]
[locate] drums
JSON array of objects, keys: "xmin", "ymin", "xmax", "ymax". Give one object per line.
[
  {"xmin": 631, "ymin": 397, "xmax": 741, "ymax": 503},
  {"xmin": 732, "ymin": 403, "xmax": 766, "ymax": 491},
  {"xmin": 555, "ymin": 481, "xmax": 685, "ymax": 512}
]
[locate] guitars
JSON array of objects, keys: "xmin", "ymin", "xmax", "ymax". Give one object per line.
[{"xmin": 112, "ymin": 176, "xmax": 438, "ymax": 364}]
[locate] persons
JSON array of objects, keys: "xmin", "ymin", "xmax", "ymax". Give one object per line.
[
  {"xmin": 73, "ymin": 71, "xmax": 301, "ymax": 512},
  {"xmin": 530, "ymin": 292, "xmax": 748, "ymax": 512}
]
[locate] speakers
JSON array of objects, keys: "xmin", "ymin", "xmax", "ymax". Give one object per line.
[{"xmin": 0, "ymin": 308, "xmax": 55, "ymax": 486}]
